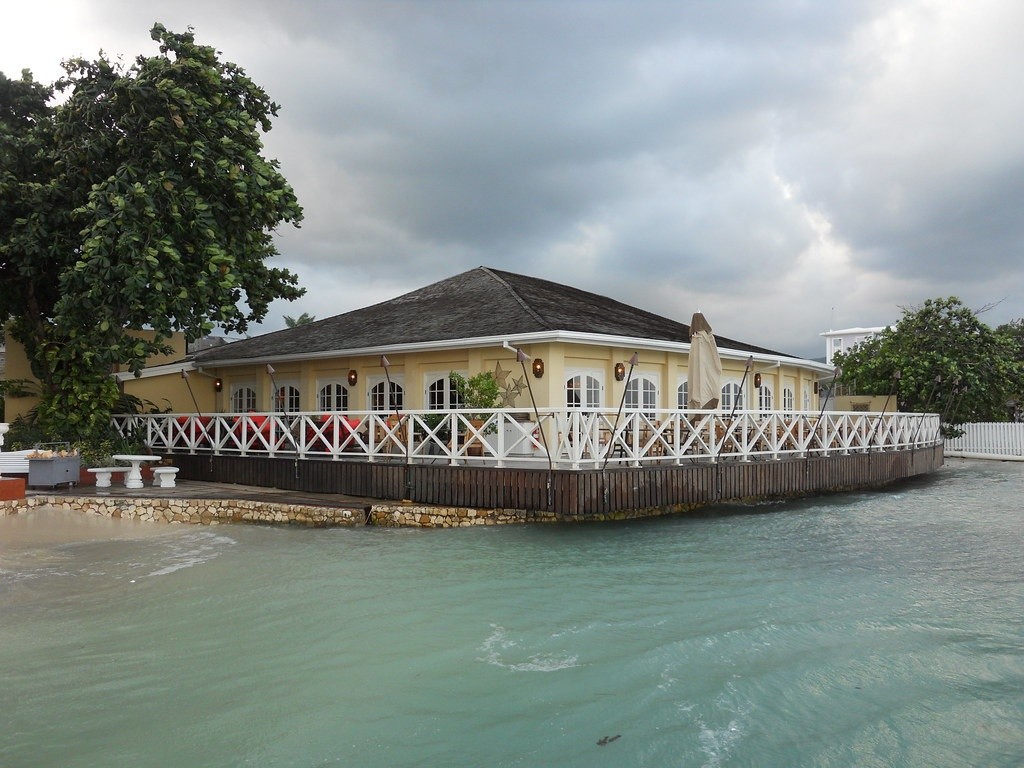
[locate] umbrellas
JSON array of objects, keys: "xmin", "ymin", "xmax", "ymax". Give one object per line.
[{"xmin": 688, "ymin": 313, "xmax": 721, "ymax": 420}]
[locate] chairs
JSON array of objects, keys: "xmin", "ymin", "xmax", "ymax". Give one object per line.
[
  {"xmin": 357, "ymin": 414, "xmax": 486, "ymax": 463},
  {"xmin": 582, "ymin": 414, "xmax": 884, "ymax": 467}
]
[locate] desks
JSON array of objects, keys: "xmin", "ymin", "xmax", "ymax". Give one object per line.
[
  {"xmin": 598, "ymin": 426, "xmax": 652, "ymax": 467},
  {"xmin": 659, "ymin": 427, "xmax": 698, "ymax": 464},
  {"xmin": 112, "ymin": 454, "xmax": 161, "ymax": 488},
  {"xmin": 727, "ymin": 427, "xmax": 757, "ymax": 461},
  {"xmin": 418, "ymin": 428, "xmax": 463, "ymax": 464}
]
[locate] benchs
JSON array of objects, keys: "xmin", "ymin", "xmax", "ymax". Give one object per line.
[
  {"xmin": 150, "ymin": 467, "xmax": 179, "ymax": 487},
  {"xmin": 87, "ymin": 467, "xmax": 143, "ymax": 488}
]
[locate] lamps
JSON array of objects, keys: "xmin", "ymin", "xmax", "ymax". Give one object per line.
[
  {"xmin": 755, "ymin": 373, "xmax": 761, "ymax": 388},
  {"xmin": 348, "ymin": 370, "xmax": 357, "ymax": 386},
  {"xmin": 615, "ymin": 363, "xmax": 625, "ymax": 381},
  {"xmin": 532, "ymin": 358, "xmax": 543, "ymax": 378},
  {"xmin": 214, "ymin": 378, "xmax": 221, "ymax": 392}
]
